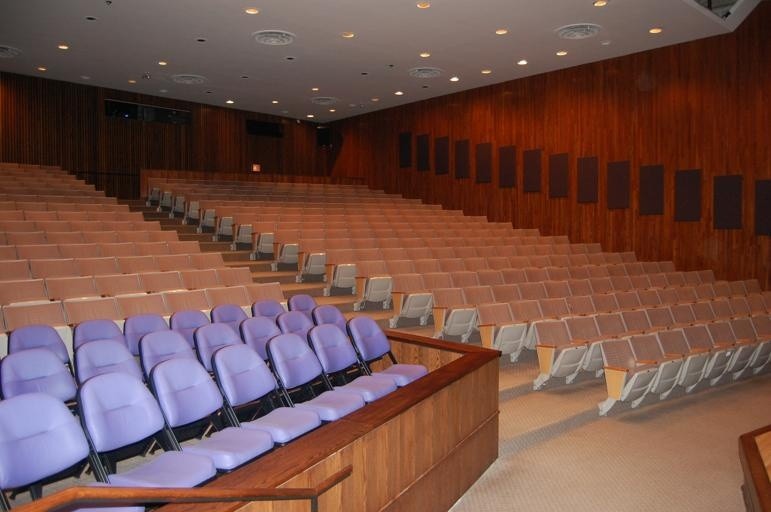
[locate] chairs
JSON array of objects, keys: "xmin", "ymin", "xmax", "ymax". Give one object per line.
[
  {"xmin": 104, "ymin": 196, "xmax": 427, "ymax": 511},
  {"xmin": 1, "ymin": 163, "xmax": 103, "ymax": 512},
  {"xmin": 427, "ymin": 203, "xmax": 767, "ymax": 416},
  {"xmin": 142, "ymin": 170, "xmax": 386, "ymax": 196}
]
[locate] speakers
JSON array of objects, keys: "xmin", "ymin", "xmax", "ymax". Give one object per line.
[{"xmin": 316, "ymin": 126, "xmax": 331, "ymax": 145}]
